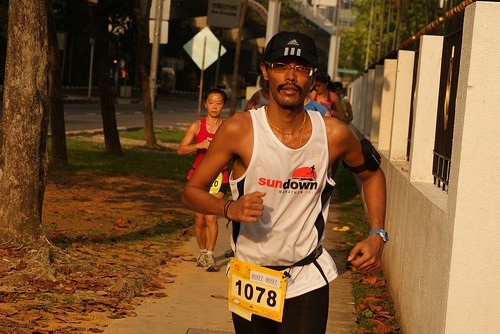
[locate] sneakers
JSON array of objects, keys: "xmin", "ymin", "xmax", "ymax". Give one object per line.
[
  {"xmin": 203, "ymin": 254, "xmax": 217, "ymax": 270},
  {"xmin": 196, "ymin": 252, "xmax": 207, "ymax": 266}
]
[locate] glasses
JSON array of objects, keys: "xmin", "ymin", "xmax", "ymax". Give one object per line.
[{"xmin": 264, "ymin": 60, "xmax": 318, "ymax": 76}]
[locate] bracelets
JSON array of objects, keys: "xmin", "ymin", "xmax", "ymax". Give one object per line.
[{"xmin": 223, "ymin": 200, "xmax": 234, "ymax": 218}]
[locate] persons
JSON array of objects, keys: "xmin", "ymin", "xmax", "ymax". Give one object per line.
[
  {"xmin": 304, "ymin": 95, "xmax": 329, "ymax": 117},
  {"xmin": 182, "ymin": 30, "xmax": 389, "ymax": 334},
  {"xmin": 245, "ymin": 73, "xmax": 270, "ymax": 111},
  {"xmin": 310, "ymin": 72, "xmax": 344, "ymax": 119},
  {"xmin": 334, "ymin": 82, "xmax": 353, "ymax": 122}
]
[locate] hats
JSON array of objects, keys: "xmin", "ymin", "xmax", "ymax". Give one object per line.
[{"xmin": 264, "ymin": 31, "xmax": 318, "ymax": 67}]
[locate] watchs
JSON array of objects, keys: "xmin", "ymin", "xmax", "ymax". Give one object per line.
[{"xmin": 368, "ymin": 229, "xmax": 389, "ymax": 243}]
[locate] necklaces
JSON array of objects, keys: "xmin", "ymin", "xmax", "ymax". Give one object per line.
[
  {"xmin": 178, "ymin": 90, "xmax": 230, "ymax": 269},
  {"xmin": 265, "ymin": 104, "xmax": 307, "ymax": 151}
]
